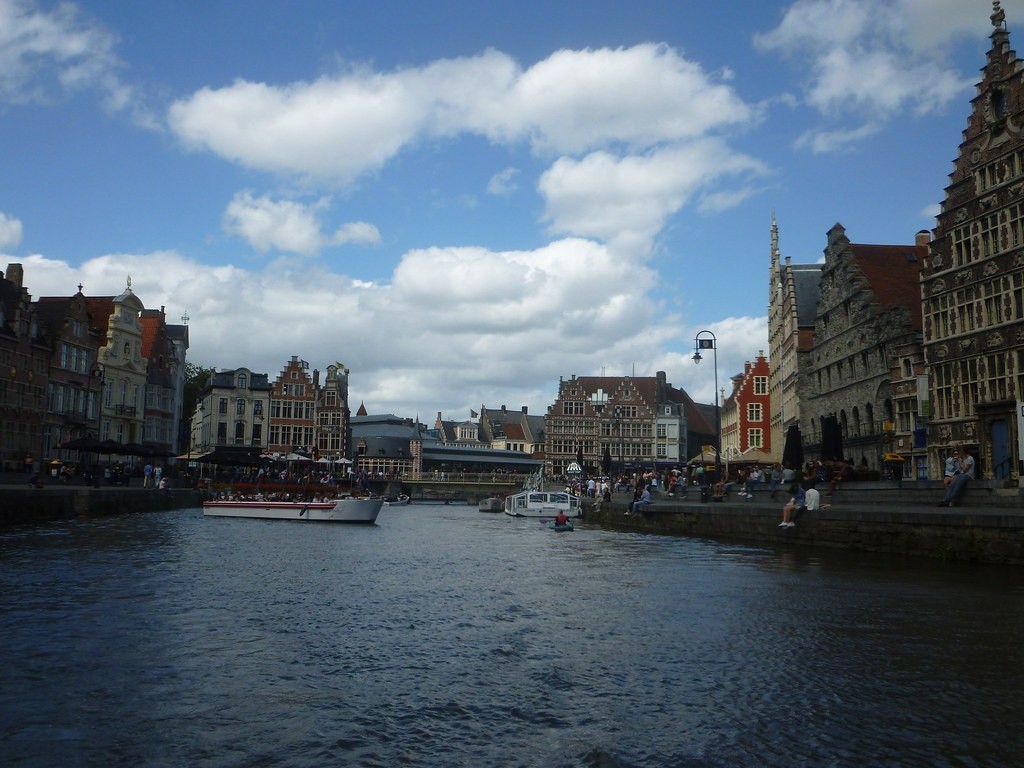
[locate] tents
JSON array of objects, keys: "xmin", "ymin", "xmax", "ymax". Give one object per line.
[
  {"xmin": 729, "ymin": 447, "xmax": 778, "ymax": 464},
  {"xmin": 687, "ymin": 452, "xmax": 729, "ymax": 472}
]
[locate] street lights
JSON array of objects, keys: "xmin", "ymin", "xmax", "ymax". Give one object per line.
[
  {"xmin": 90, "ymin": 361, "xmax": 105, "ymax": 472},
  {"xmin": 691, "ymin": 330, "xmax": 721, "ymax": 483}
]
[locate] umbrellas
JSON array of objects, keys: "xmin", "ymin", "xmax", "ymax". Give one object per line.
[
  {"xmin": 281, "ymin": 453, "xmax": 311, "ymax": 469},
  {"xmin": 62, "ymin": 437, "xmax": 102, "ymax": 464},
  {"xmin": 335, "ymin": 458, "xmax": 353, "ymax": 473},
  {"xmin": 100, "ymin": 439, "xmax": 124, "ymax": 464},
  {"xmin": 176, "ymin": 451, "xmax": 204, "ymax": 469},
  {"xmin": 140, "ymin": 446, "xmax": 161, "ymax": 464},
  {"xmin": 117, "ymin": 443, "xmax": 142, "ymax": 465},
  {"xmin": 316, "ymin": 457, "xmax": 331, "ymax": 463}
]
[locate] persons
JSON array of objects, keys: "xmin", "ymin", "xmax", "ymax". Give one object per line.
[
  {"xmin": 460, "ymin": 468, "xmax": 467, "ymax": 477},
  {"xmin": 25, "ymin": 454, "xmax": 142, "ymax": 487},
  {"xmin": 553, "ymin": 456, "xmax": 867, "ymax": 529},
  {"xmin": 940, "ymin": 448, "xmax": 975, "ymax": 507},
  {"xmin": 490, "ymin": 466, "xmax": 519, "ymax": 483},
  {"xmin": 137, "ymin": 464, "xmax": 406, "ymax": 503},
  {"xmin": 432, "ymin": 469, "xmax": 445, "ymax": 482}
]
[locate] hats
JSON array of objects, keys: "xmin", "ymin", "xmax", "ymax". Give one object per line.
[{"xmin": 754, "ymin": 466, "xmax": 759, "ymax": 468}]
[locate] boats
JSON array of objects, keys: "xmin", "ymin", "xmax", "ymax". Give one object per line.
[
  {"xmin": 479, "ymin": 495, "xmax": 502, "ymax": 511},
  {"xmin": 202, "ymin": 495, "xmax": 383, "ymax": 523},
  {"xmin": 548, "ymin": 519, "xmax": 574, "ymax": 531},
  {"xmin": 346, "ymin": 494, "xmax": 409, "ymax": 506},
  {"xmin": 504, "ymin": 489, "xmax": 583, "ymax": 517}
]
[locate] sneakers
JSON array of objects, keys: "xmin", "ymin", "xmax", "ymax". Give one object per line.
[
  {"xmin": 778, "ymin": 522, "xmax": 787, "ymax": 527},
  {"xmin": 747, "ymin": 494, "xmax": 752, "ymax": 498},
  {"xmin": 742, "ymin": 493, "xmax": 747, "ymax": 496},
  {"xmin": 737, "ymin": 492, "xmax": 743, "ymax": 495},
  {"xmin": 788, "ymin": 522, "xmax": 794, "ymax": 527},
  {"xmin": 594, "ymin": 510, "xmax": 597, "ymax": 511},
  {"xmin": 597, "ymin": 509, "xmax": 600, "ymax": 511},
  {"xmin": 741, "ymin": 488, "xmax": 745, "ymax": 491},
  {"xmin": 624, "ymin": 512, "xmax": 629, "ymax": 515}
]
